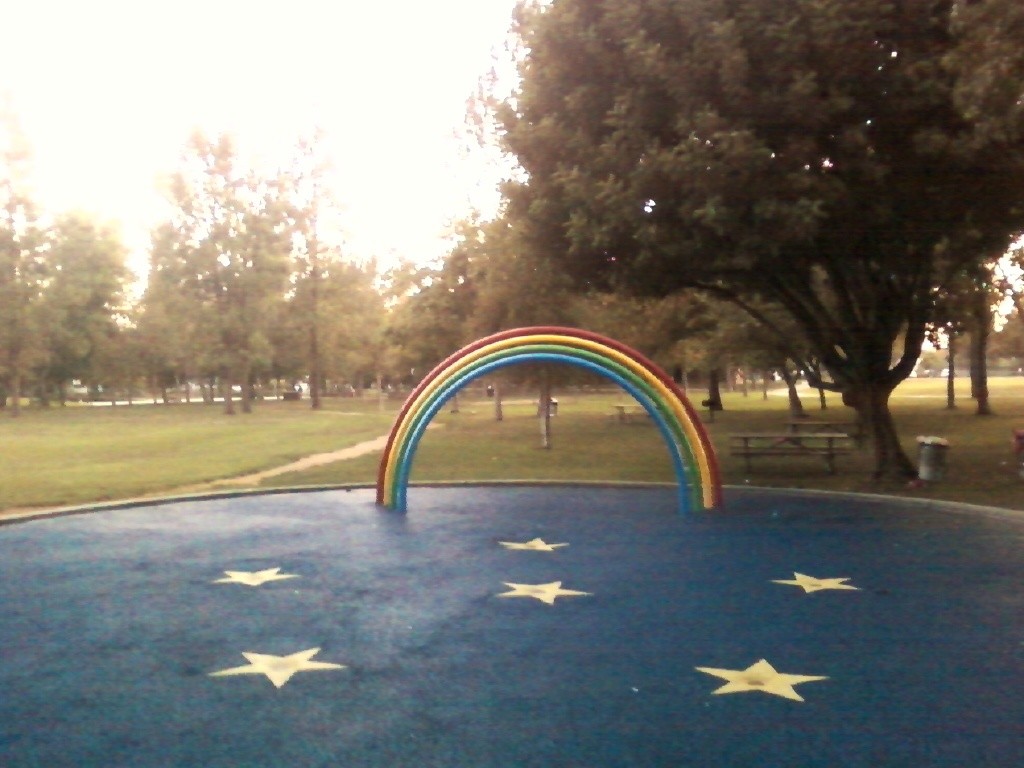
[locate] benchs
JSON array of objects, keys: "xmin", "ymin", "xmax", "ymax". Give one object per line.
[
  {"xmin": 782, "ymin": 420, "xmax": 868, "ymax": 448},
  {"xmin": 727, "ymin": 431, "xmax": 851, "ymax": 475},
  {"xmin": 610, "ymin": 405, "xmax": 653, "ymax": 424}
]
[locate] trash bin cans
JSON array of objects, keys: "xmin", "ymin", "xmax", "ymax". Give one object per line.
[
  {"xmin": 549, "ymin": 398, "xmax": 558, "ymax": 417},
  {"xmin": 918, "ymin": 437, "xmax": 950, "ymax": 481}
]
